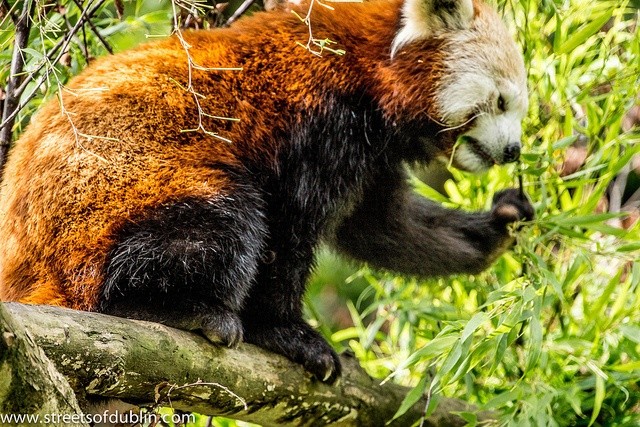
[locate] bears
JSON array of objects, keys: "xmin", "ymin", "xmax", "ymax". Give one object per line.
[{"xmin": 0, "ymin": 0, "xmax": 534, "ymax": 385}]
